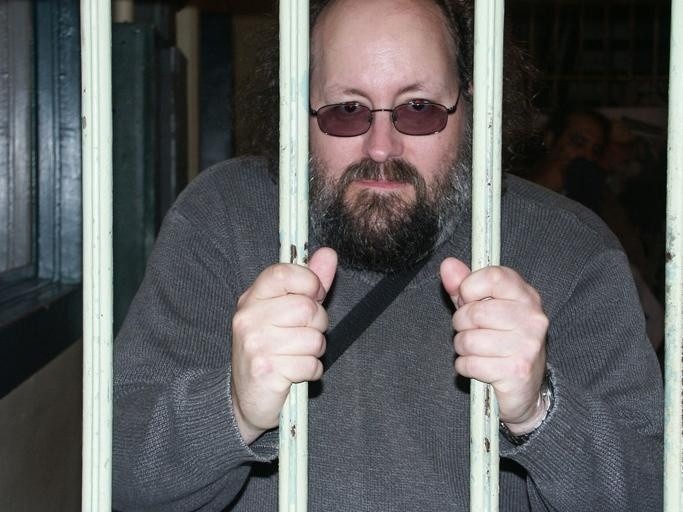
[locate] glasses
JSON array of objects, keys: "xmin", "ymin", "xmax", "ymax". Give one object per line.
[{"xmin": 309, "ymin": 89, "xmax": 461, "ymax": 137}]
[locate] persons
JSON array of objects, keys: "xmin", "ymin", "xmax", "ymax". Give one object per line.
[
  {"xmin": 506, "ymin": 103, "xmax": 662, "ymax": 350},
  {"xmin": 64, "ymin": 0, "xmax": 663, "ymax": 511}
]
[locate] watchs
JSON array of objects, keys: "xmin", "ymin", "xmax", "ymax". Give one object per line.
[{"xmin": 499, "ymin": 379, "xmax": 555, "ymax": 446}]
